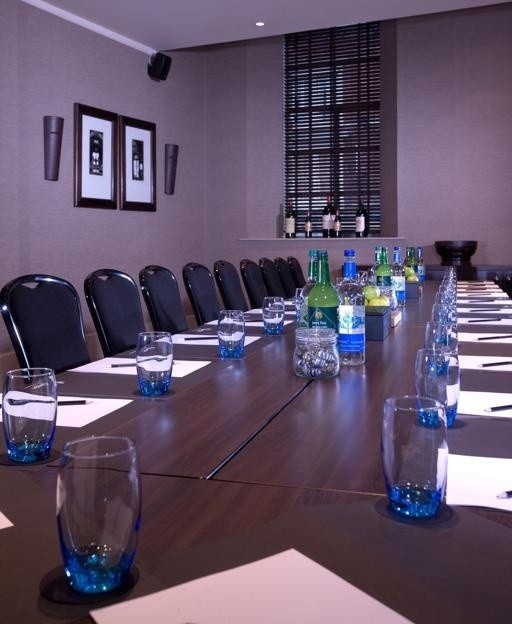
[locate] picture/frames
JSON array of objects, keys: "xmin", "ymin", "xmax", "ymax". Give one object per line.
[
  {"xmin": 119, "ymin": 114, "xmax": 157, "ymax": 212},
  {"xmin": 73, "ymin": 104, "xmax": 119, "ymax": 209}
]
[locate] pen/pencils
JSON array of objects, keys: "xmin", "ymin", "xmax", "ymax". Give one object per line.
[
  {"xmin": 0, "ymin": 399, "xmax": 94, "ymax": 408},
  {"xmin": 177, "ymin": 337, "xmax": 219, "ymax": 341},
  {"xmin": 476, "ymin": 361, "xmax": 512, "ymax": 367},
  {"xmin": 465, "ymin": 318, "xmax": 501, "ymax": 322},
  {"xmin": 483, "ymin": 405, "xmax": 512, "ymax": 412},
  {"xmin": 233, "ymin": 319, "xmax": 263, "ymax": 322},
  {"xmin": 496, "ymin": 491, "xmax": 512, "ymax": 499},
  {"xmin": 467, "ymin": 308, "xmax": 500, "ymax": 312},
  {"xmin": 469, "ymin": 300, "xmax": 494, "ymax": 302},
  {"xmin": 473, "ymin": 335, "xmax": 512, "ymax": 340},
  {"xmin": 467, "ymin": 294, "xmax": 492, "ymax": 296},
  {"xmin": 102, "ymin": 356, "xmax": 176, "ymax": 368}
]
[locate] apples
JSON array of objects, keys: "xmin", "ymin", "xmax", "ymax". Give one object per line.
[
  {"xmin": 363, "ymin": 285, "xmax": 390, "ymax": 306},
  {"xmin": 405, "ymin": 267, "xmax": 419, "ymax": 283}
]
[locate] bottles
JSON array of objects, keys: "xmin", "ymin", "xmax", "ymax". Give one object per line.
[
  {"xmin": 322, "ymin": 195, "xmax": 342, "ymax": 236},
  {"xmin": 339, "ymin": 249, "xmax": 364, "ymax": 365},
  {"xmin": 292, "ymin": 330, "xmax": 341, "ymax": 380},
  {"xmin": 298, "ymin": 249, "xmax": 320, "ymax": 328},
  {"xmin": 354, "ymin": 194, "xmax": 368, "ymax": 238},
  {"xmin": 374, "ymin": 244, "xmax": 426, "ymax": 307},
  {"xmin": 308, "ymin": 247, "xmax": 338, "ymax": 345},
  {"xmin": 285, "ymin": 198, "xmax": 296, "ymax": 238},
  {"xmin": 303, "ymin": 210, "xmax": 314, "ymax": 239}
]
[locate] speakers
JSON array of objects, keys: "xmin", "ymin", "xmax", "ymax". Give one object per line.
[{"xmin": 148, "ymin": 51, "xmax": 173, "ymax": 82}]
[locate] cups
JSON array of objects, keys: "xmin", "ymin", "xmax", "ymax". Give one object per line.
[
  {"xmin": 261, "ymin": 296, "xmax": 286, "ymax": 335},
  {"xmin": 136, "ymin": 329, "xmax": 174, "ymax": 396},
  {"xmin": 4, "ymin": 366, "xmax": 58, "ymax": 464},
  {"xmin": 218, "ymin": 308, "xmax": 248, "ymax": 359},
  {"xmin": 296, "ymin": 287, "xmax": 305, "ymax": 307},
  {"xmin": 412, "ymin": 347, "xmax": 461, "ymax": 429},
  {"xmin": 56, "ymin": 435, "xmax": 142, "ymax": 594},
  {"xmin": 424, "ymin": 263, "xmax": 458, "ymax": 344},
  {"xmin": 380, "ymin": 394, "xmax": 449, "ymax": 520}
]
